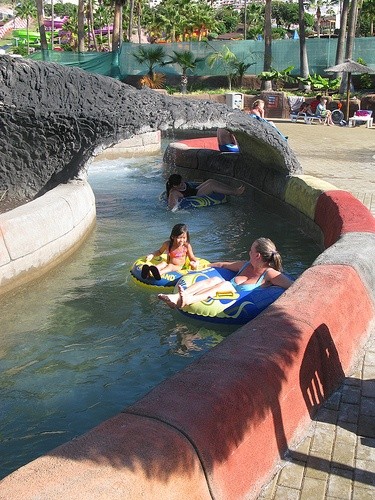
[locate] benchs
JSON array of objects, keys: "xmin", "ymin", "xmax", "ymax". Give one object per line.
[{"xmin": 287, "ymin": 95, "xmax": 332, "ymax": 124}]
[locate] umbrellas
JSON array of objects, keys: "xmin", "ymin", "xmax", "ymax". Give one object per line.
[{"xmin": 323, "ymin": 59, "xmax": 375, "ymax": 122}]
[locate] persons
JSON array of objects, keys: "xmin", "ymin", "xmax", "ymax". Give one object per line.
[
  {"xmin": 158, "ymin": 238, "xmax": 294, "ymax": 308},
  {"xmin": 300, "ymin": 95, "xmax": 334, "ymax": 126},
  {"xmin": 218, "ymin": 128, "xmax": 239, "ymax": 151},
  {"xmin": 141, "ymin": 224, "xmax": 196, "ymax": 280},
  {"xmin": 241, "ymin": 100, "xmax": 288, "ymax": 140},
  {"xmin": 166, "ymin": 174, "xmax": 246, "ymax": 208}
]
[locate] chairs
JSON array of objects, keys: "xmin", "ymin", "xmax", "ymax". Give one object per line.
[{"xmin": 348, "ymin": 109, "xmax": 373, "ymax": 129}]
